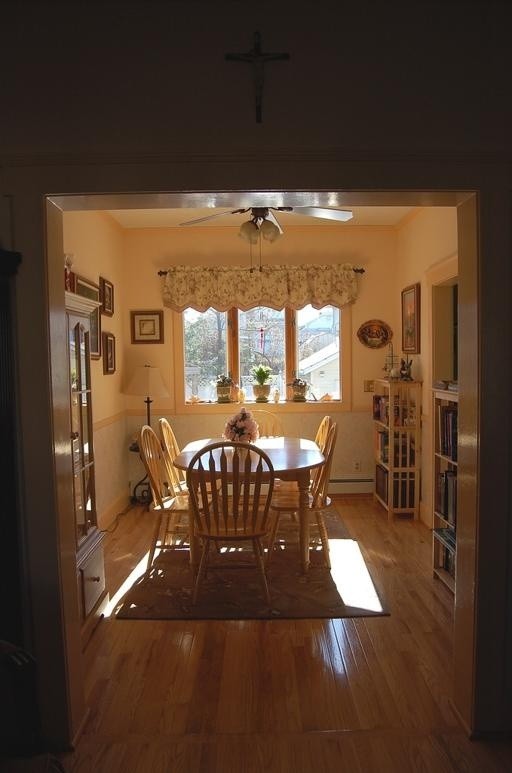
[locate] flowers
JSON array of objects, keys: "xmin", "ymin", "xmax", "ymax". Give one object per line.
[{"xmin": 220, "ymin": 407, "xmax": 262, "ymax": 445}]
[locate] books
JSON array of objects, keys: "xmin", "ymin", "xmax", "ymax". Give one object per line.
[
  {"xmin": 433, "ymin": 378, "xmax": 458, "ymax": 582},
  {"xmin": 371, "ymin": 392, "xmax": 416, "ymax": 467}
]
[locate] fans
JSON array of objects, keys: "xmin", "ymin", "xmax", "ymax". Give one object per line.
[{"xmin": 177, "ymin": 205, "xmax": 353, "ymax": 233}]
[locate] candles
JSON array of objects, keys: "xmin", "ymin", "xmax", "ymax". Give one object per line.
[{"xmin": 146, "ymin": 366, "xmax": 151, "ymax": 398}]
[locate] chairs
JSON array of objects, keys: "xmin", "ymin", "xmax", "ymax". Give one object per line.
[
  {"xmin": 136, "ymin": 424, "xmax": 212, "ymax": 566},
  {"xmin": 270, "ymin": 414, "xmax": 333, "ymax": 528},
  {"xmin": 157, "ymin": 416, "xmax": 221, "ymax": 553},
  {"xmin": 263, "ymin": 422, "xmax": 338, "ymax": 573},
  {"xmin": 249, "ymin": 409, "xmax": 284, "ymax": 438},
  {"xmin": 185, "ymin": 441, "xmax": 277, "ymax": 607}
]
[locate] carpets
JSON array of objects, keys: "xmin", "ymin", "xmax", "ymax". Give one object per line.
[{"xmin": 116, "ymin": 498, "xmax": 390, "ymax": 619}]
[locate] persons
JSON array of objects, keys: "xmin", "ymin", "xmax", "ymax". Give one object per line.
[{"xmin": 0, "ymin": 637, "xmax": 68, "ymax": 773}]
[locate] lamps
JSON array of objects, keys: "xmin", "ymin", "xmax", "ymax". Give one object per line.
[{"xmin": 232, "ymin": 207, "xmax": 293, "ymax": 272}]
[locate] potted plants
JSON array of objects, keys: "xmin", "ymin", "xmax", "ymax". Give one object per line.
[
  {"xmin": 287, "ymin": 378, "xmax": 308, "ymax": 401},
  {"xmin": 250, "ymin": 365, "xmax": 272, "ymax": 403},
  {"xmin": 216, "ymin": 375, "xmax": 232, "ymax": 403}
]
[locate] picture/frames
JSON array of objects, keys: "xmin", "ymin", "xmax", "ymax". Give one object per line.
[
  {"xmin": 102, "ymin": 331, "xmax": 116, "ymax": 375},
  {"xmin": 400, "ymin": 282, "xmax": 420, "ymax": 355},
  {"xmin": 71, "ymin": 272, "xmax": 102, "ymax": 360},
  {"xmin": 99, "ymin": 275, "xmax": 114, "ymax": 317},
  {"xmin": 130, "ymin": 310, "xmax": 165, "ymax": 344},
  {"xmin": 357, "ymin": 320, "xmax": 393, "ymax": 349}
]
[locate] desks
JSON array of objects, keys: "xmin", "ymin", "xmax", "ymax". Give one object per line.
[
  {"xmin": 171, "ymin": 434, "xmax": 326, "ymax": 574},
  {"xmin": 129, "ymin": 442, "xmax": 165, "ymax": 505}
]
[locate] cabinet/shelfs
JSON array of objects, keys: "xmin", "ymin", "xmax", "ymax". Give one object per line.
[
  {"xmin": 371, "ymin": 378, "xmax": 422, "ymax": 522},
  {"xmin": 431, "ymin": 386, "xmax": 459, "ymax": 608},
  {"xmin": 65, "ymin": 289, "xmax": 111, "ymax": 684}
]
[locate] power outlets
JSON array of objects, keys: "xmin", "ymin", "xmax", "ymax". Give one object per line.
[{"xmin": 352, "ymin": 460, "xmax": 361, "ymax": 472}]
